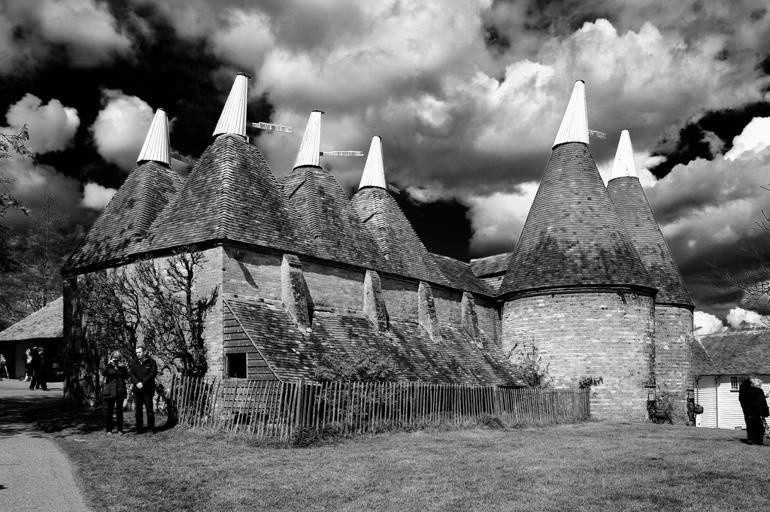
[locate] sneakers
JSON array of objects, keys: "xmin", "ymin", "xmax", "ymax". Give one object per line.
[{"xmin": 108, "ymin": 431, "xmax": 123, "ymax": 436}]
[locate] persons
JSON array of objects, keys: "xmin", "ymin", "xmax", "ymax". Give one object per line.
[
  {"xmin": 23, "ymin": 344, "xmax": 50, "ymax": 391},
  {"xmin": 129, "ymin": 345, "xmax": 158, "ymax": 434},
  {"xmin": 739, "ymin": 377, "xmax": 769, "ymax": 445},
  {"xmin": 101, "ymin": 350, "xmax": 129, "ymax": 436}
]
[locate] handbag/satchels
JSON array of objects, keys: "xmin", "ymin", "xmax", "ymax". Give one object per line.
[{"xmin": 103, "ymin": 379, "xmax": 117, "ymax": 399}]
